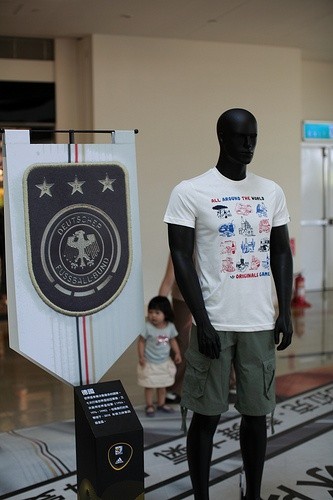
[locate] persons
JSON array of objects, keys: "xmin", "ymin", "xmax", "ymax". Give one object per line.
[
  {"xmin": 161, "ymin": 109, "xmax": 297, "ymax": 500},
  {"xmin": 135, "ymin": 295, "xmax": 182, "ymax": 417},
  {"xmin": 156, "ymin": 250, "xmax": 192, "ymax": 405}
]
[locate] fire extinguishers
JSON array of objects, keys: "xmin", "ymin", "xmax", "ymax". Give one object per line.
[{"xmin": 292, "ymin": 273, "xmax": 305, "ymax": 308}]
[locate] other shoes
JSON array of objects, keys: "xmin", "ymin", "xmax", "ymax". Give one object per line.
[
  {"xmin": 157, "ymin": 404, "xmax": 173, "ymax": 412},
  {"xmin": 145, "ymin": 405, "xmax": 155, "ymax": 416}
]
